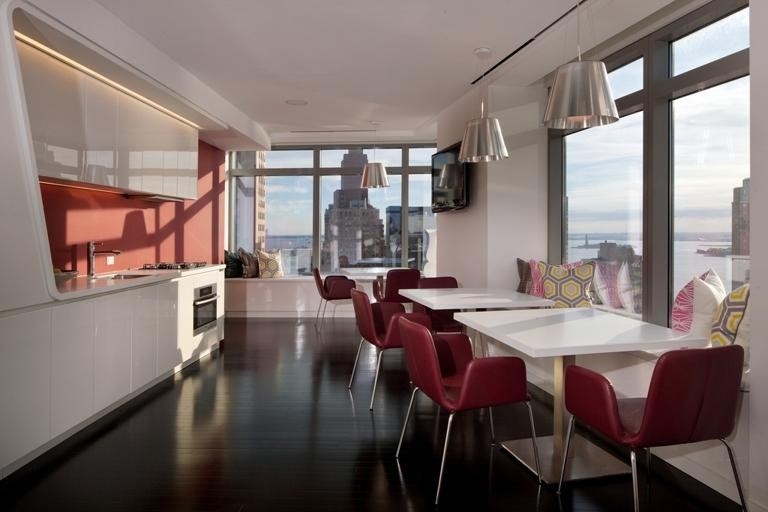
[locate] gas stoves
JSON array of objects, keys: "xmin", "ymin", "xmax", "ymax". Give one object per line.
[{"xmin": 140, "ymin": 261, "xmax": 206, "ymax": 269}]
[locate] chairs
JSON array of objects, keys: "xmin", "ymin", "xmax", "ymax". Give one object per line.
[
  {"xmin": 556, "ymin": 344, "xmax": 747, "ymax": 512},
  {"xmin": 417, "ymin": 277, "xmax": 463, "ymax": 332},
  {"xmin": 347, "ymin": 287, "xmax": 432, "ymax": 412},
  {"xmin": 373, "ymin": 269, "xmax": 420, "ymax": 303},
  {"xmin": 395, "ymin": 316, "xmax": 542, "ymax": 504},
  {"xmin": 313, "ymin": 268, "xmax": 356, "ymax": 334}
]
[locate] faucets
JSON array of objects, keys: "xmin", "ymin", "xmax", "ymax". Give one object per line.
[{"xmin": 86, "ymin": 240, "xmax": 121, "ymax": 276}]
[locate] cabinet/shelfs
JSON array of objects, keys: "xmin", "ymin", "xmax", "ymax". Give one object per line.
[
  {"xmin": 16, "ymin": 39, "xmax": 198, "ymax": 201},
  {"xmin": 0, "ymin": 274, "xmax": 180, "ymax": 481}
]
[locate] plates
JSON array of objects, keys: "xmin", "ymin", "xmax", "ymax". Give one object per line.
[{"xmin": 54, "ymin": 272, "xmax": 77, "ymax": 277}]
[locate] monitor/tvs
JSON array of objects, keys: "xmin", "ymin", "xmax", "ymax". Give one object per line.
[
  {"xmin": 195, "ymin": 298, "xmax": 214, "ymax": 334},
  {"xmin": 431, "ymin": 140, "xmax": 469, "ymax": 213}
]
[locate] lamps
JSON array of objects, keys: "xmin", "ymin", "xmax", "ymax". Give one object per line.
[
  {"xmin": 359, "ymin": 122, "xmax": 390, "ymax": 188},
  {"xmin": 458, "ymin": 47, "xmax": 510, "ymax": 162},
  {"xmin": 542, "ymin": 1, "xmax": 620, "ymax": 129}
]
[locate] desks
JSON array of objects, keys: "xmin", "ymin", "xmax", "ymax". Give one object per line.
[
  {"xmin": 340, "ymin": 268, "xmax": 412, "ymax": 297},
  {"xmin": 453, "ymin": 306, "xmax": 707, "ymax": 484},
  {"xmin": 398, "ymin": 287, "xmax": 556, "ymax": 358}
]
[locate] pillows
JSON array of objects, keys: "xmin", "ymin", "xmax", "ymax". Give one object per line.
[
  {"xmin": 224, "ymin": 249, "xmax": 243, "ymax": 278},
  {"xmin": 256, "ymin": 249, "xmax": 284, "ymax": 278},
  {"xmin": 530, "ymin": 259, "xmax": 583, "ymax": 297},
  {"xmin": 516, "ymin": 258, "xmax": 533, "ymax": 295},
  {"xmin": 239, "ymin": 247, "xmax": 261, "ymax": 278},
  {"xmin": 711, "ymin": 283, "xmax": 751, "ymax": 366},
  {"xmin": 592, "ymin": 261, "xmax": 637, "ymax": 314},
  {"xmin": 538, "ymin": 261, "xmax": 597, "ymax": 309},
  {"xmin": 672, "ymin": 267, "xmax": 727, "ymax": 350}
]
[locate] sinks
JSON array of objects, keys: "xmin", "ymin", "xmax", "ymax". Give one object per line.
[{"xmin": 92, "ymin": 274, "xmax": 153, "ymax": 280}]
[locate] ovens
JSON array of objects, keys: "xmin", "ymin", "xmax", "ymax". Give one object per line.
[{"xmin": 193, "ymin": 282, "xmax": 217, "ymax": 337}]
